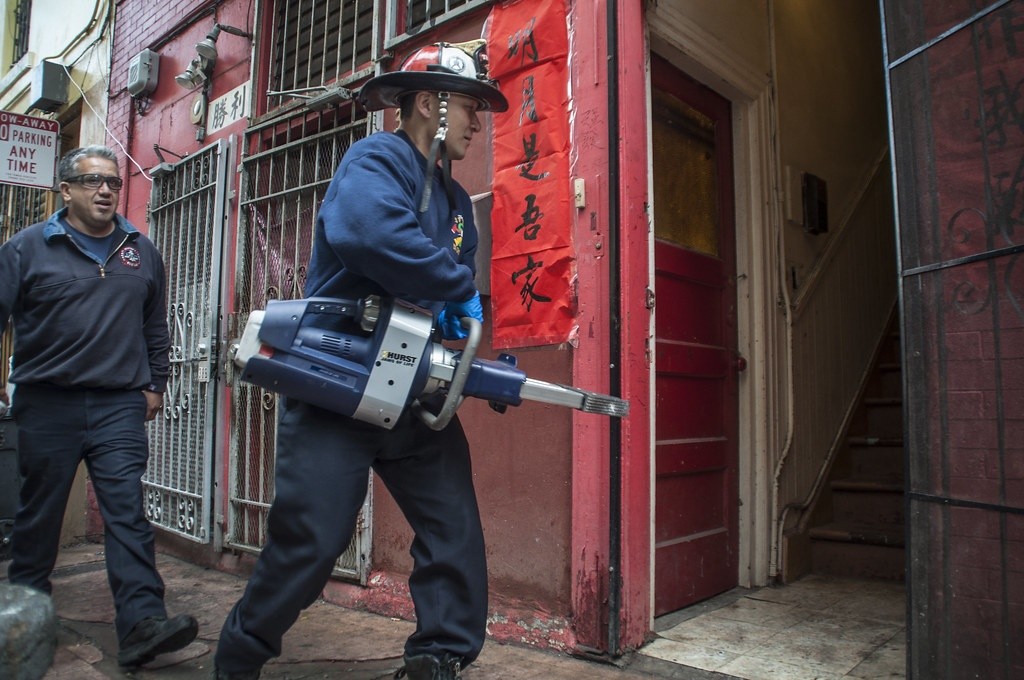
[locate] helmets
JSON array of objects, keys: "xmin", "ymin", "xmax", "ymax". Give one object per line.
[{"xmin": 355, "ymin": 41, "xmax": 508, "ymax": 113}]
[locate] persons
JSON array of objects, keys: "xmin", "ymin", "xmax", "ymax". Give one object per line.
[
  {"xmin": 211, "ymin": 38, "xmax": 489, "ymax": 680},
  {"xmin": 0, "ymin": 146, "xmax": 201, "ymax": 674}
]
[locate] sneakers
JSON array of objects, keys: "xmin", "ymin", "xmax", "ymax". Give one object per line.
[{"xmin": 116, "ymin": 614, "xmax": 199, "ymax": 666}]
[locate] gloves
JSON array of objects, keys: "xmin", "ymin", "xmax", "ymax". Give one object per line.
[{"xmin": 437, "ymin": 290, "xmax": 482, "ymax": 341}]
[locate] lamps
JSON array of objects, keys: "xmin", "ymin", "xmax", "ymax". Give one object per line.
[
  {"xmin": 174, "ymin": 58, "xmax": 206, "ymax": 92},
  {"xmin": 195, "ymin": 24, "xmax": 253, "ymax": 61}
]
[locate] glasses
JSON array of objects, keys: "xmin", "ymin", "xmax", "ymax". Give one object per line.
[{"xmin": 64, "ymin": 173, "xmax": 123, "ymax": 190}]
